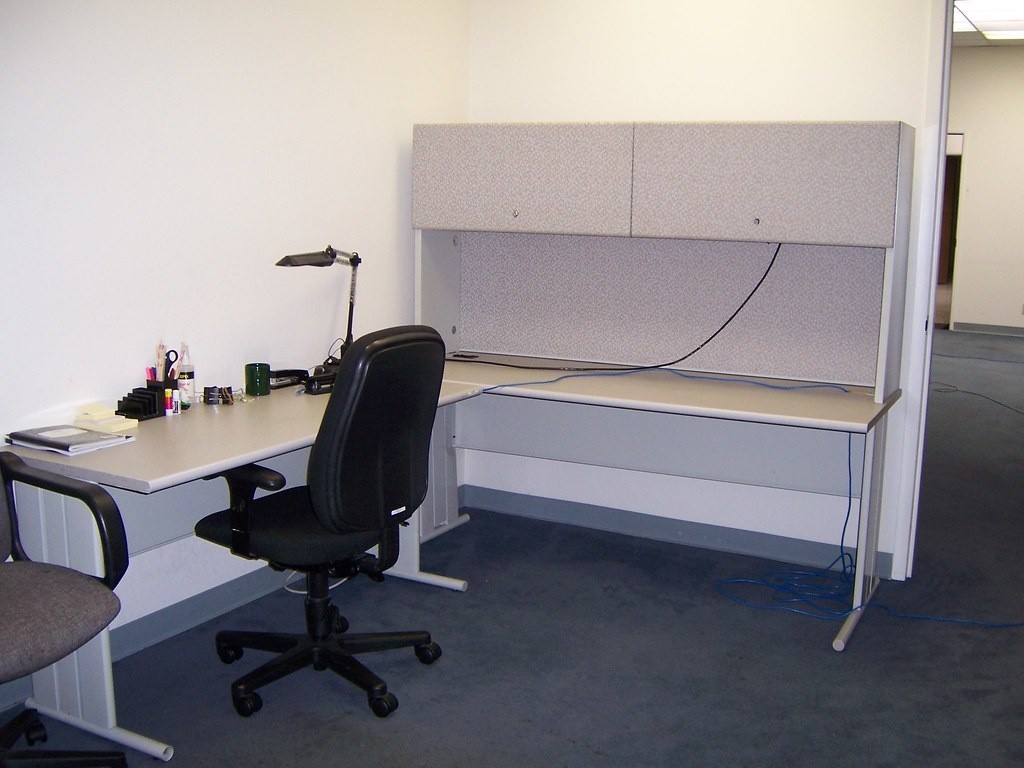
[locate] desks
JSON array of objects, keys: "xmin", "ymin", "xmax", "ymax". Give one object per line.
[
  {"xmin": 0, "ymin": 385, "xmax": 480, "ymax": 763},
  {"xmin": 439, "ymin": 353, "xmax": 901, "ymax": 651}
]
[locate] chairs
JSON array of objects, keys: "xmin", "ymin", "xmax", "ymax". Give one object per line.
[
  {"xmin": 194, "ymin": 325, "xmax": 446, "ymax": 720},
  {"xmin": 0, "ymin": 452, "xmax": 130, "ymax": 768}
]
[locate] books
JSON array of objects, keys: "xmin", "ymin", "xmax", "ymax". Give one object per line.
[{"xmin": 7, "ymin": 405, "xmax": 139, "ymax": 457}]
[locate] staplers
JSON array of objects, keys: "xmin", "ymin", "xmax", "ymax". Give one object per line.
[
  {"xmin": 269, "ymin": 369, "xmax": 310, "ymax": 390},
  {"xmin": 305, "ymin": 373, "xmax": 338, "ymax": 395}
]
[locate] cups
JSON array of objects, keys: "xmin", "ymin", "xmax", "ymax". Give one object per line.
[
  {"xmin": 178, "ymin": 365, "xmax": 195, "ymax": 405},
  {"xmin": 245, "ymin": 363, "xmax": 270, "ymax": 396}
]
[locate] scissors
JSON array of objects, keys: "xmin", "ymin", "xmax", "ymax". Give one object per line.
[{"xmin": 165, "ymin": 350, "xmax": 179, "ymax": 378}]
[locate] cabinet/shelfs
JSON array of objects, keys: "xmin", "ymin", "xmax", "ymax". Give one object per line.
[{"xmin": 411, "ymin": 121, "xmax": 917, "ymax": 404}]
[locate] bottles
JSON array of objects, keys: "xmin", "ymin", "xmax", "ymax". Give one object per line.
[{"xmin": 172, "ymin": 390, "xmax": 181, "ymax": 415}]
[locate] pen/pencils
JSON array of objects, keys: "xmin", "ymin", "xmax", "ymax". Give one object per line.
[{"xmin": 144, "ymin": 340, "xmax": 188, "ymax": 381}]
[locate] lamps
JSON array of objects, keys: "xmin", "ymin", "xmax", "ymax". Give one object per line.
[{"xmin": 275, "ymin": 246, "xmax": 361, "ymax": 395}]
